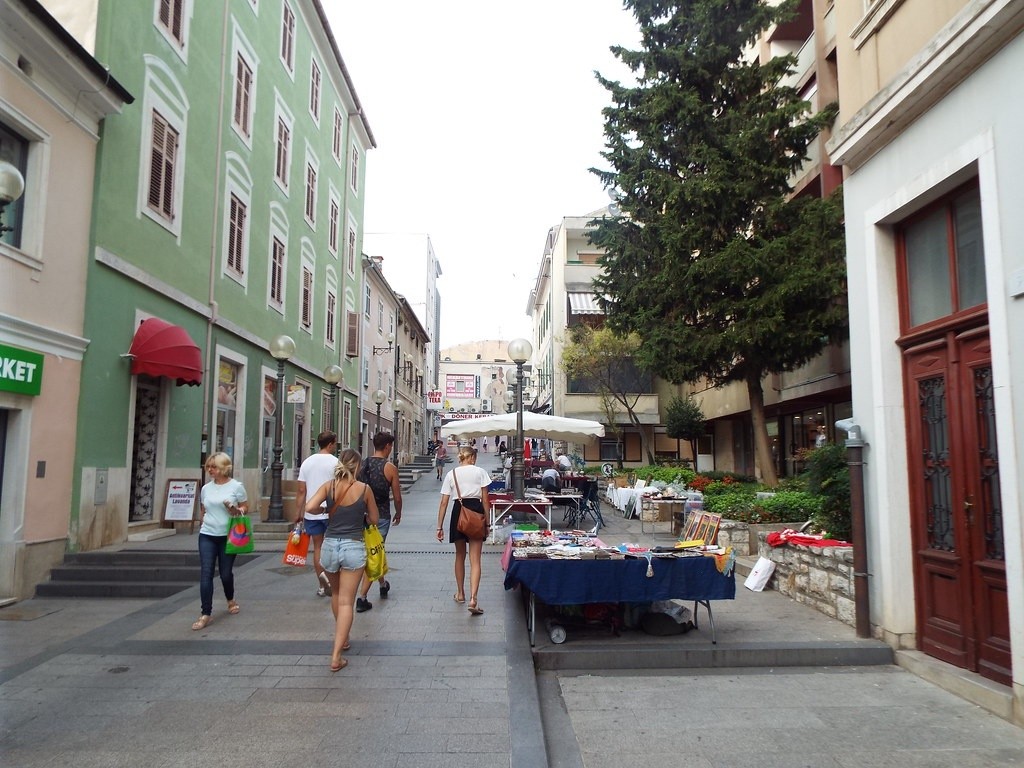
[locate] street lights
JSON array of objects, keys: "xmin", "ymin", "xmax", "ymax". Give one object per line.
[
  {"xmin": 391, "ymin": 399, "xmax": 404, "ymax": 472},
  {"xmin": 507, "ymin": 337, "xmax": 534, "ymax": 499},
  {"xmin": 268, "ymin": 335, "xmax": 297, "ymax": 524},
  {"xmin": 324, "ymin": 365, "xmax": 345, "ymax": 460},
  {"xmin": 503, "ymin": 390, "xmax": 516, "ymax": 413},
  {"xmin": 506, "ymin": 366, "xmax": 526, "ymax": 414},
  {"xmin": 372, "ymin": 389, "xmax": 387, "ymax": 436}
]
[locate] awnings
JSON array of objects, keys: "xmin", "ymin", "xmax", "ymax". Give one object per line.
[{"xmin": 129, "ymin": 318, "xmax": 203, "ymax": 387}]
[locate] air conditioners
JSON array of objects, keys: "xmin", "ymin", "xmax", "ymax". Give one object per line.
[{"xmin": 449, "ymin": 398, "xmax": 492, "ymax": 414}]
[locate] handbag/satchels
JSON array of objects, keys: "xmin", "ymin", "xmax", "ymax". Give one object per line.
[
  {"xmin": 431, "ymin": 454, "xmax": 437, "ymax": 467},
  {"xmin": 224, "ymin": 508, "xmax": 256, "ymax": 554},
  {"xmin": 364, "ymin": 524, "xmax": 388, "ymax": 581},
  {"xmin": 283, "ymin": 529, "xmax": 310, "ymax": 567},
  {"xmin": 457, "ymin": 505, "xmax": 486, "ymax": 539}
]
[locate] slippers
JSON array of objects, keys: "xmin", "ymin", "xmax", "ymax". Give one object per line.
[
  {"xmin": 468, "ymin": 601, "xmax": 483, "ymax": 614},
  {"xmin": 454, "ymin": 592, "xmax": 466, "ymax": 603}
]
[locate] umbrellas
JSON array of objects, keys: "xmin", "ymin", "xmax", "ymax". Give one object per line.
[{"xmin": 441, "ymin": 411, "xmax": 606, "ymax": 442}]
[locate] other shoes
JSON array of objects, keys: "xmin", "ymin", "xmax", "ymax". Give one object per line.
[
  {"xmin": 317, "ymin": 587, "xmax": 332, "ymax": 597},
  {"xmin": 440, "ymin": 477, "xmax": 442, "ymax": 481},
  {"xmin": 552, "ymin": 505, "xmax": 558, "ymax": 510},
  {"xmin": 437, "ymin": 475, "xmax": 439, "ymax": 479}
]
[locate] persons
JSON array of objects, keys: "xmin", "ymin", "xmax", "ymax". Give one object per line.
[
  {"xmin": 192, "ymin": 452, "xmax": 248, "ymax": 630},
  {"xmin": 436, "ymin": 446, "xmax": 493, "ymax": 613},
  {"xmin": 306, "ymin": 450, "xmax": 381, "ymax": 670},
  {"xmin": 291, "ymin": 431, "xmax": 402, "ymax": 612},
  {"xmin": 436, "ymin": 435, "xmax": 571, "ymax": 510}
]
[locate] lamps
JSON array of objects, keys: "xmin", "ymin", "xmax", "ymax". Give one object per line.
[
  {"xmin": 394, "ymin": 354, "xmax": 413, "ymax": 374},
  {"xmin": 523, "ymin": 359, "xmax": 552, "ymax": 400},
  {"xmin": 405, "ymin": 369, "xmax": 424, "ymax": 386},
  {"xmin": 374, "ymin": 333, "xmax": 394, "ymax": 356},
  {"xmin": 421, "ymin": 384, "xmax": 437, "ymax": 396}
]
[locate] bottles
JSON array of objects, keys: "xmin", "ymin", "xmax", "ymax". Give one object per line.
[{"xmin": 291, "ymin": 521, "xmax": 301, "ymax": 544}]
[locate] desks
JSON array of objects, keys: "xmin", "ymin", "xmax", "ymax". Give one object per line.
[
  {"xmin": 641, "ymin": 496, "xmax": 704, "ymax": 541},
  {"xmin": 488, "ymin": 477, "xmax": 591, "ymax": 545},
  {"xmin": 501, "ymin": 531, "xmax": 736, "ymax": 643}
]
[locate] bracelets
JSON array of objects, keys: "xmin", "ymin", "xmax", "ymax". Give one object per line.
[
  {"xmin": 437, "ymin": 529, "xmax": 442, "ymax": 531},
  {"xmin": 322, "ymin": 507, "xmax": 327, "ymax": 514}
]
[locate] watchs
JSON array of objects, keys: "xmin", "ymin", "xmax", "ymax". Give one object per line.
[{"xmin": 487, "ymin": 524, "xmax": 490, "ymax": 527}]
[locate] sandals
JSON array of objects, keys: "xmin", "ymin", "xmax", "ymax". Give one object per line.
[
  {"xmin": 331, "ymin": 657, "xmax": 349, "ymax": 671},
  {"xmin": 228, "ymin": 600, "xmax": 240, "ymax": 614},
  {"xmin": 191, "ymin": 616, "xmax": 214, "ymax": 630},
  {"xmin": 342, "ymin": 640, "xmax": 351, "ymax": 649}
]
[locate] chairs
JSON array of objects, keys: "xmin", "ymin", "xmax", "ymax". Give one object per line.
[{"xmin": 563, "ymin": 480, "xmax": 606, "ymax": 529}]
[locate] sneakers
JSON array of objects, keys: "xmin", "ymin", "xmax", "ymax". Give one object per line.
[
  {"xmin": 356, "ymin": 597, "xmax": 373, "ymax": 612},
  {"xmin": 380, "ymin": 581, "xmax": 390, "ymax": 599}
]
